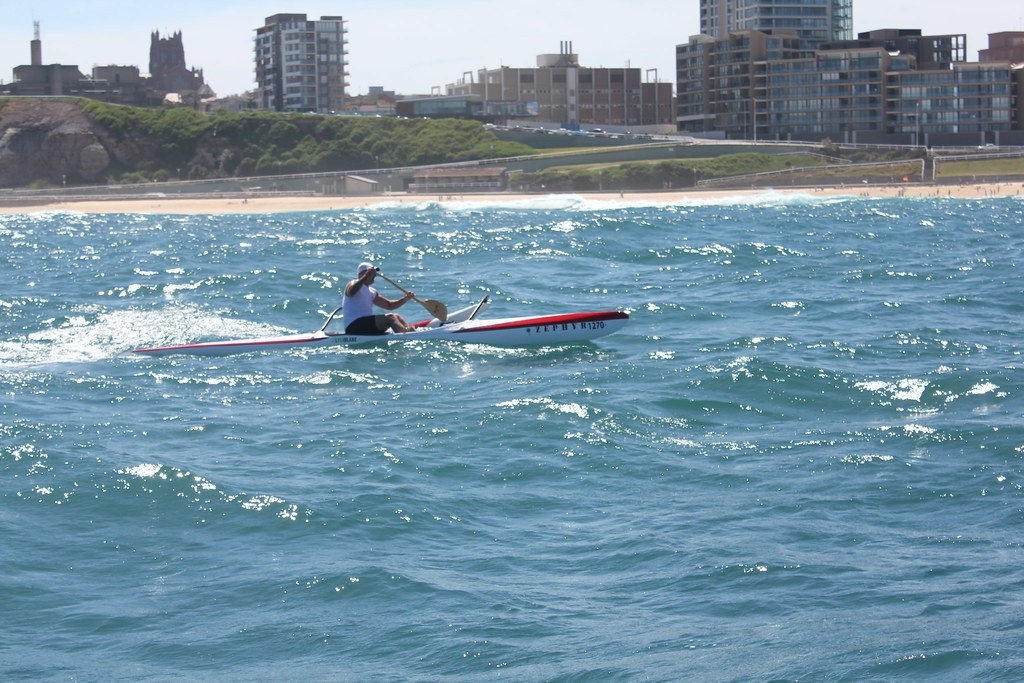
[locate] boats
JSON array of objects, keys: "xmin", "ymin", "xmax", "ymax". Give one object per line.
[{"xmin": 132, "ymin": 311, "xmax": 631, "ymax": 357}]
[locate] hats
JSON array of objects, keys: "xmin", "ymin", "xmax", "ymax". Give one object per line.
[{"xmin": 356, "ymin": 261, "xmax": 380, "ymax": 275}]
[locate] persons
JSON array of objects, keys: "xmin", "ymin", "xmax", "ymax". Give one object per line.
[
  {"xmin": 243, "ymin": 176, "xmax": 1020, "ymax": 214},
  {"xmin": 342, "ymin": 259, "xmax": 417, "ymax": 333}
]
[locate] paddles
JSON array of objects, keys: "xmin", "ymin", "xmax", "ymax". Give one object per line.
[{"xmin": 368, "ymin": 268, "xmax": 448, "ymax": 323}]
